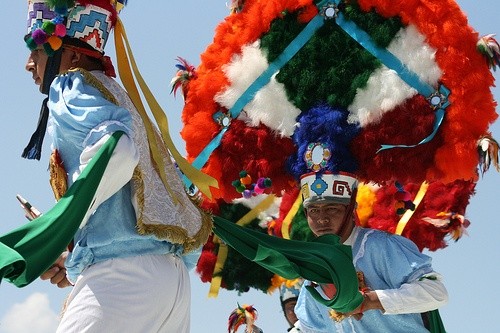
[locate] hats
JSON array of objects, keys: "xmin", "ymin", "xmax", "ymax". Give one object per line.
[
  {"xmin": 277, "ymin": 281, "xmax": 302, "ymax": 304},
  {"xmin": 24, "ymin": 0, "xmax": 122, "ymax": 58},
  {"xmin": 298, "ymin": 169, "xmax": 357, "ymax": 208}
]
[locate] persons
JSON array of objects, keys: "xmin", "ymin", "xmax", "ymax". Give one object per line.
[
  {"xmin": 24, "ymin": 0, "xmax": 190, "ymax": 333},
  {"xmin": 280, "ymin": 185, "xmax": 450, "ymax": 333}
]
